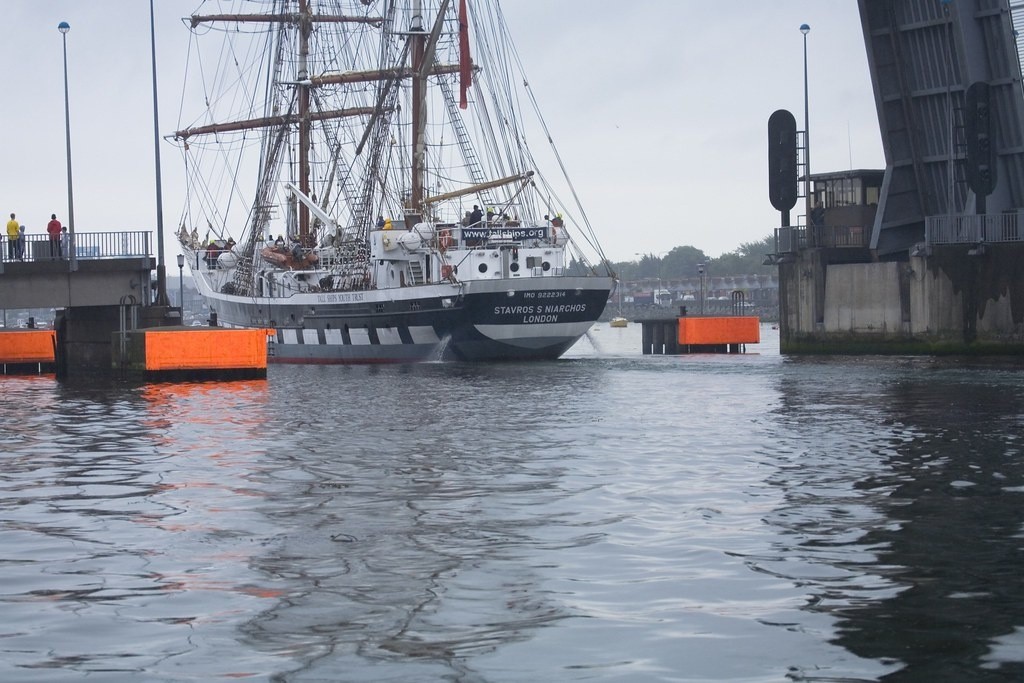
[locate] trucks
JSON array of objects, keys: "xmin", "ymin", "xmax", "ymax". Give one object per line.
[{"xmin": 634, "ymin": 290, "xmax": 672, "ymax": 310}]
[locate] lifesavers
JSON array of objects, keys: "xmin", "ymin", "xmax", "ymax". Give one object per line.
[{"xmin": 440, "ymin": 228, "xmax": 452, "ymax": 248}]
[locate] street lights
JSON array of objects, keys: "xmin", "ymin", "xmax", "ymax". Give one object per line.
[
  {"xmin": 57, "ymin": 22, "xmax": 78, "ymax": 272},
  {"xmin": 697, "ymin": 262, "xmax": 705, "ymax": 316},
  {"xmin": 176, "ymin": 254, "xmax": 185, "ymax": 327},
  {"xmin": 799, "ymin": 22, "xmax": 814, "ymax": 249}
]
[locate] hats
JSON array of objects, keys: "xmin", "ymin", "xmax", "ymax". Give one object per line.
[
  {"xmin": 210, "ymin": 240, "xmax": 214, "ymax": 244},
  {"xmin": 488, "ymin": 208, "xmax": 492, "ymax": 212},
  {"xmin": 556, "ymin": 213, "xmax": 562, "ymax": 218}
]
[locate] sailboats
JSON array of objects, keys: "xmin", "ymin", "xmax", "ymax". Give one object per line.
[{"xmin": 163, "ymin": 0, "xmax": 617, "ymax": 363}]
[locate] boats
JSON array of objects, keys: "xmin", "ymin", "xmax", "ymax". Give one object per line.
[
  {"xmin": 610, "ymin": 317, "xmax": 627, "ymax": 326},
  {"xmin": 411, "ymin": 222, "xmax": 434, "ymax": 241},
  {"xmin": 397, "ymin": 232, "xmax": 421, "ymax": 252}
]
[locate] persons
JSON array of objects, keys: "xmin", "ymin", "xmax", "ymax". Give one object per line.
[
  {"xmin": 204, "ymin": 237, "xmax": 236, "ymax": 269},
  {"xmin": 376, "ymin": 215, "xmax": 392, "ymax": 231},
  {"xmin": 266, "ymin": 234, "xmax": 286, "ymax": 248},
  {"xmin": 7, "ymin": 213, "xmax": 69, "ymax": 262},
  {"xmin": 461, "ymin": 205, "xmax": 563, "ymax": 227},
  {"xmin": 810, "ymin": 201, "xmax": 826, "ymax": 246}
]
